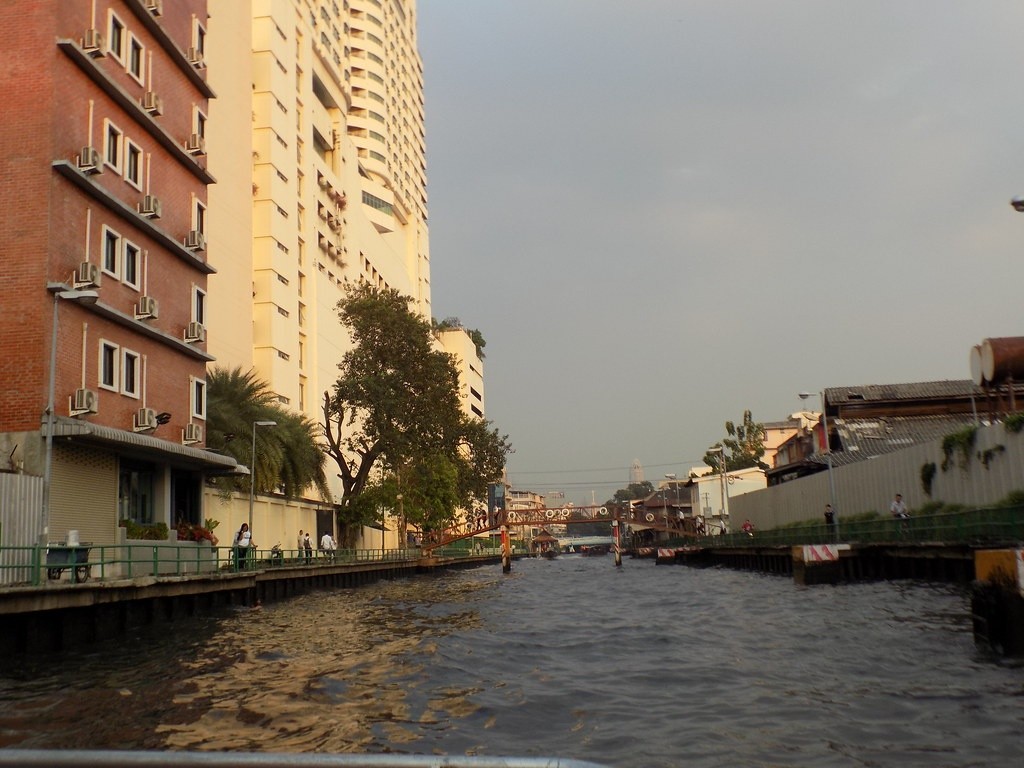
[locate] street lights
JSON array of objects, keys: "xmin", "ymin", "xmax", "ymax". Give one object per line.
[
  {"xmin": 665, "ymin": 474, "xmax": 681, "ymax": 543},
  {"xmin": 707, "ymin": 445, "xmax": 734, "ymax": 550},
  {"xmin": 39, "ymin": 290, "xmax": 102, "ymax": 573},
  {"xmin": 246, "ymin": 420, "xmax": 278, "ymax": 545},
  {"xmin": 798, "ymin": 391, "xmax": 841, "ymax": 543}
]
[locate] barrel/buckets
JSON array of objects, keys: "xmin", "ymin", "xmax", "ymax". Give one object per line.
[{"xmin": 66, "ymin": 529, "xmax": 80, "ymax": 546}]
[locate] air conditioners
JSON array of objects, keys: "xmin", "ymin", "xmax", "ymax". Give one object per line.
[
  {"xmin": 146, "ymin": 0, "xmax": 162, "ymax": 17},
  {"xmin": 132, "ymin": 408, "xmax": 158, "ymax": 433},
  {"xmin": 184, "ymin": 231, "xmax": 205, "ymax": 251},
  {"xmin": 185, "ymin": 134, "xmax": 206, "ymax": 157},
  {"xmin": 76, "ymin": 146, "xmax": 104, "ymax": 176},
  {"xmin": 73, "ymin": 262, "xmax": 101, "ymax": 290},
  {"xmin": 184, "ymin": 322, "xmax": 204, "ymax": 344},
  {"xmin": 139, "ymin": 91, "xmax": 163, "ymax": 116},
  {"xmin": 137, "ymin": 195, "xmax": 161, "ymax": 220},
  {"xmin": 181, "ymin": 423, "xmax": 203, "ymax": 446},
  {"xmin": 133, "ymin": 296, "xmax": 158, "ymax": 321},
  {"xmin": 185, "ymin": 47, "xmax": 204, "ymax": 70},
  {"xmin": 68, "ymin": 388, "xmax": 99, "ymax": 419},
  {"xmin": 80, "ymin": 29, "xmax": 106, "ymax": 59}
]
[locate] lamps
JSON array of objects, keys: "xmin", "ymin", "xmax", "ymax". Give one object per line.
[{"xmin": 152, "ymin": 412, "xmax": 171, "ymax": 435}]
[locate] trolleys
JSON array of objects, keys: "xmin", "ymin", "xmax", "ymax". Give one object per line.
[{"xmin": 46, "ymin": 541, "xmax": 94, "ymax": 583}]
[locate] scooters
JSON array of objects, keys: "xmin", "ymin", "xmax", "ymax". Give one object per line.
[{"xmin": 268, "ymin": 542, "xmax": 283, "ymax": 566}]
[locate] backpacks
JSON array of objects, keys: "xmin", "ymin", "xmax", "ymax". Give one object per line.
[{"xmin": 303, "ymin": 538, "xmax": 309, "ymax": 546}]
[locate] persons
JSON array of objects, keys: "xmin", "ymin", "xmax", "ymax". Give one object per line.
[
  {"xmin": 742, "ymin": 520, "xmax": 752, "ymax": 532},
  {"xmin": 304, "ymin": 533, "xmax": 313, "ymax": 557},
  {"xmin": 822, "ymin": 505, "xmax": 835, "ymax": 533},
  {"xmin": 477, "ymin": 510, "xmax": 486, "ymax": 531},
  {"xmin": 321, "ymin": 531, "xmax": 337, "ymax": 557},
  {"xmin": 234, "ymin": 523, "xmax": 258, "ymax": 570},
  {"xmin": 696, "ymin": 515, "xmax": 704, "ymax": 534},
  {"xmin": 415, "ymin": 535, "xmax": 421, "ymax": 548},
  {"xmin": 719, "ymin": 518, "xmax": 724, "ymax": 534},
  {"xmin": 475, "ymin": 542, "xmax": 484, "ymax": 555},
  {"xmin": 512, "ymin": 544, "xmax": 516, "ymax": 554},
  {"xmin": 890, "ymin": 493, "xmax": 908, "ymax": 519},
  {"xmin": 297, "ymin": 530, "xmax": 304, "ymax": 557},
  {"xmin": 678, "ymin": 509, "xmax": 687, "ymax": 531}
]
